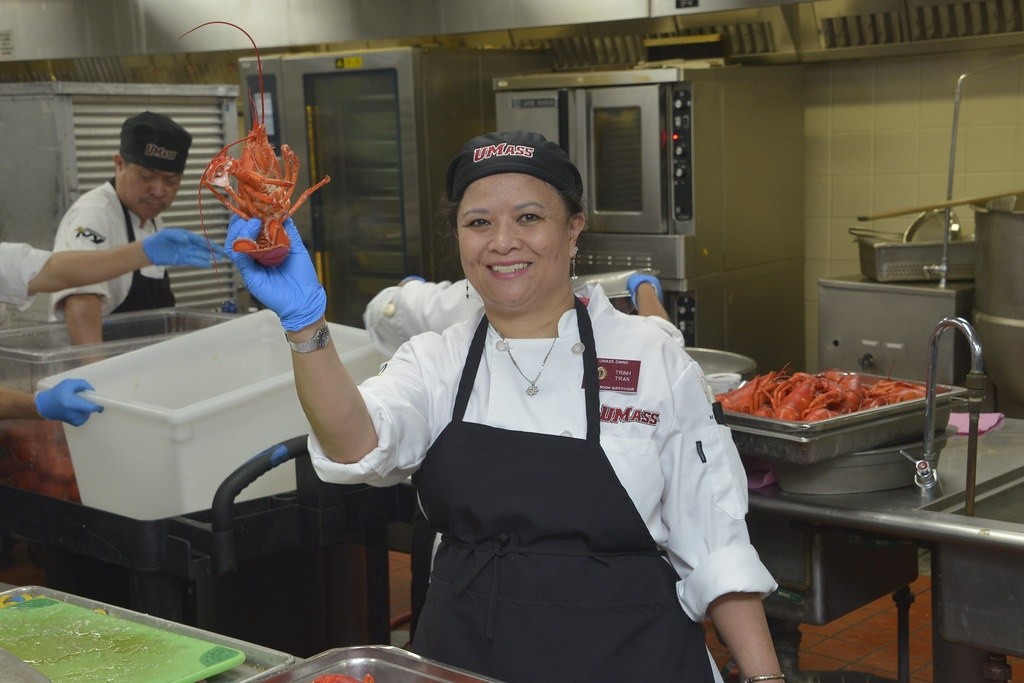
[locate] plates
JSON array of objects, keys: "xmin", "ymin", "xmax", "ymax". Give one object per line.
[{"xmin": 903, "ymin": 207, "xmax": 961, "ymax": 242}]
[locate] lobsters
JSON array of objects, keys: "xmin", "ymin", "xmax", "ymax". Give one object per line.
[
  {"xmin": 312, "ymin": 673, "xmax": 376, "ymax": 683},
  {"xmin": 713, "ymin": 361, "xmax": 951, "ymax": 423},
  {"xmin": 176, "ymin": 20, "xmax": 330, "ymax": 291}
]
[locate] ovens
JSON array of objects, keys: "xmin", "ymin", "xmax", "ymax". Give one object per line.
[
  {"xmin": 493, "ymin": 68, "xmax": 807, "ymax": 374},
  {"xmin": 240, "ymin": 47, "xmax": 561, "ymax": 327}
]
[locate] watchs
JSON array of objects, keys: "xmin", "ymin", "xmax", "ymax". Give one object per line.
[{"xmin": 284, "ymin": 323, "xmax": 332, "ymax": 354}]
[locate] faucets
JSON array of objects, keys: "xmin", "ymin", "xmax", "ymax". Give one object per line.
[{"xmin": 921, "ymin": 311, "xmax": 987, "ymax": 470}]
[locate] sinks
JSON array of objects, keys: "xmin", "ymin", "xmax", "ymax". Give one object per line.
[{"xmin": 912, "ymin": 460, "xmax": 1024, "ymax": 658}]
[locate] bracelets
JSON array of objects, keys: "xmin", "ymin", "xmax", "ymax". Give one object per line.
[{"xmin": 744, "ymin": 674, "xmax": 785, "ymax": 683}]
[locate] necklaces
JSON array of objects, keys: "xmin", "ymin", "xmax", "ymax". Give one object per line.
[{"xmin": 494, "ymin": 327, "xmax": 557, "ymax": 397}]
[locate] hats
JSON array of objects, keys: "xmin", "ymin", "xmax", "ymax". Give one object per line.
[
  {"xmin": 120, "ymin": 109, "xmax": 193, "ymax": 175},
  {"xmin": 445, "ymin": 129, "xmax": 584, "ymax": 204}
]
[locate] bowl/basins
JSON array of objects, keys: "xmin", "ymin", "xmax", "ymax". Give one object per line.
[{"xmin": 684, "ymin": 348, "xmax": 757, "ymax": 379}]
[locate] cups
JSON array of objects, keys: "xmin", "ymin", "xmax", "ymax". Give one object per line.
[{"xmin": 707, "ymin": 373, "xmax": 741, "ymax": 395}]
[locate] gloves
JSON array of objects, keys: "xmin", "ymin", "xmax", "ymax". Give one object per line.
[
  {"xmin": 626, "ymin": 270, "xmax": 663, "ymax": 311},
  {"xmin": 400, "ymin": 274, "xmax": 426, "ymax": 284},
  {"xmin": 221, "ymin": 211, "xmax": 329, "ymax": 334},
  {"xmin": 143, "ymin": 226, "xmax": 227, "ymax": 269},
  {"xmin": 33, "ymin": 378, "xmax": 104, "ymax": 427}
]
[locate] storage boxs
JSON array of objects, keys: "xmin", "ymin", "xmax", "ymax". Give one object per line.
[
  {"xmin": 0, "ymin": 307, "xmax": 247, "ymax": 502},
  {"xmin": 857, "ymin": 236, "xmax": 977, "ymax": 282},
  {"xmin": 36, "ymin": 307, "xmax": 396, "ymax": 522}
]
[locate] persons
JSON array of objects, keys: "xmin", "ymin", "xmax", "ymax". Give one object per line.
[
  {"xmin": 0, "ymin": 228, "xmax": 224, "ymax": 426},
  {"xmin": 46, "ymin": 112, "xmax": 192, "ymax": 367},
  {"xmin": 364, "ymin": 271, "xmax": 685, "ymax": 362},
  {"xmin": 226, "ymin": 131, "xmax": 786, "ymax": 683}
]
